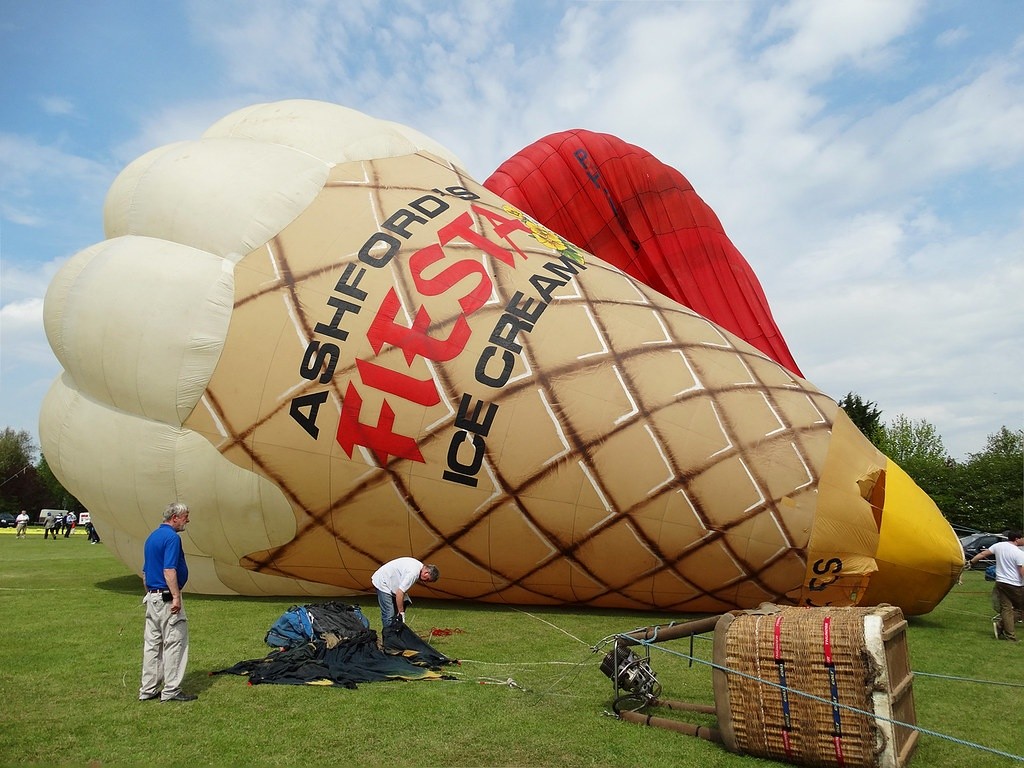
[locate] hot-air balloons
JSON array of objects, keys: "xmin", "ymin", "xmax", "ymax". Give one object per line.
[
  {"xmin": 38, "ymin": 98, "xmax": 966, "ymax": 617},
  {"xmin": 481, "ymin": 128, "xmax": 807, "ymax": 378}
]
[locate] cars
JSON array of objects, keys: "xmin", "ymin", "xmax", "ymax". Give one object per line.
[
  {"xmin": 0, "ymin": 512, "xmax": 17, "ymax": 528},
  {"xmin": 958, "ymin": 534, "xmax": 1009, "ymax": 563}
]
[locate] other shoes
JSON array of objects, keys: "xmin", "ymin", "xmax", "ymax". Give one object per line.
[{"xmin": 958, "ymin": 581, "xmax": 963, "ymax": 585}]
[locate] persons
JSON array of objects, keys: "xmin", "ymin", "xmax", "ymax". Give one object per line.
[
  {"xmin": 371, "ymin": 556, "xmax": 439, "ymax": 647},
  {"xmin": 14, "ymin": 509, "xmax": 100, "ymax": 546},
  {"xmin": 964, "ymin": 529, "xmax": 1024, "ymax": 644},
  {"xmin": 140, "ymin": 502, "xmax": 199, "ymax": 704}
]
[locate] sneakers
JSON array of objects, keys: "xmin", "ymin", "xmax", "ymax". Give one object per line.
[
  {"xmin": 993, "ymin": 622, "xmax": 1000, "ymax": 639},
  {"xmin": 91, "ymin": 542, "xmax": 95, "ymax": 544}
]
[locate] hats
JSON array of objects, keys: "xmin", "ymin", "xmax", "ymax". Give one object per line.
[{"xmin": 69, "ymin": 512, "xmax": 73, "ymax": 515}]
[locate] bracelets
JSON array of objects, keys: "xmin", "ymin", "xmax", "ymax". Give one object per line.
[{"xmin": 969, "ymin": 560, "xmax": 974, "ymax": 565}]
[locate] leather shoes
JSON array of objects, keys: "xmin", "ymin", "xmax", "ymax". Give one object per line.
[
  {"xmin": 172, "ymin": 691, "xmax": 197, "ymax": 702},
  {"xmin": 139, "ymin": 693, "xmax": 161, "ymax": 699}
]
